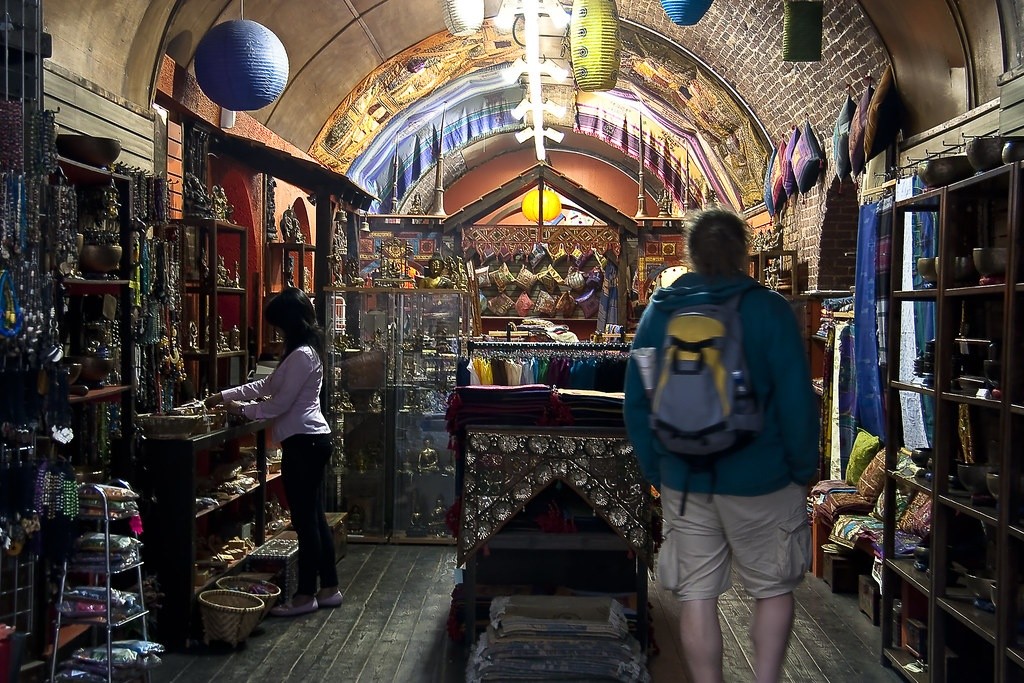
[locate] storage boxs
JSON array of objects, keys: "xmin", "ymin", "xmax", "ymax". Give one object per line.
[
  {"xmin": 325, "ymin": 511, "xmax": 350, "ymax": 565},
  {"xmin": 822, "ymin": 553, "xmax": 862, "ymax": 596},
  {"xmin": 243, "ymin": 538, "xmax": 298, "ymax": 604},
  {"xmin": 857, "ymin": 574, "xmax": 881, "ymax": 627}
]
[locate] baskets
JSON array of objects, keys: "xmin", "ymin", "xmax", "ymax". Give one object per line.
[{"xmin": 198, "ymin": 575, "xmax": 282, "ymax": 650}]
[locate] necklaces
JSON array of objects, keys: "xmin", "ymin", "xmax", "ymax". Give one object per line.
[
  {"xmin": 26, "ymin": 466, "xmax": 80, "ymax": 521},
  {"xmin": 81, "ymin": 163, "xmax": 186, "ymax": 469},
  {"xmin": 0, "ymin": 97, "xmax": 78, "ymax": 369}
]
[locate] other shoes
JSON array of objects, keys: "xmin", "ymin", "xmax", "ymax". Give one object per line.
[
  {"xmin": 319, "ymin": 589, "xmax": 343, "ymax": 607},
  {"xmin": 270, "ymin": 597, "xmax": 319, "ymax": 616}
]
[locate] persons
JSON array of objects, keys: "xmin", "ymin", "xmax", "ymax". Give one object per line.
[
  {"xmin": 620, "ymin": 212, "xmax": 822, "ymax": 683},
  {"xmin": 339, "ymin": 393, "xmax": 439, "ymax": 473},
  {"xmin": 204, "ymin": 288, "xmax": 343, "ymax": 615},
  {"xmin": 419, "ymin": 252, "xmax": 454, "ymax": 308},
  {"xmin": 338, "ymin": 500, "xmax": 446, "ymax": 531}
]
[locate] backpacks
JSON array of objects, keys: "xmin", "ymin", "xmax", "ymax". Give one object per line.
[{"xmin": 646, "ymin": 284, "xmax": 772, "ymax": 458}]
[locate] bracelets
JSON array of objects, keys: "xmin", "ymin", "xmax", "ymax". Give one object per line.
[{"xmin": 241, "ymin": 407, "xmax": 244, "ymax": 417}]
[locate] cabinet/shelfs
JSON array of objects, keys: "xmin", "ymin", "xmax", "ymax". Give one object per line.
[
  {"xmin": 139, "ymin": 418, "xmax": 296, "ymax": 653},
  {"xmin": 48, "ymin": 478, "xmax": 152, "ymax": 683},
  {"xmin": 880, "ymin": 158, "xmax": 1024, "ymax": 683},
  {"xmin": 320, "ymin": 286, "xmax": 469, "ymax": 547},
  {"xmin": 52, "ymin": 151, "xmax": 138, "ymax": 482},
  {"xmin": 260, "ymin": 241, "xmax": 318, "ymax": 359},
  {"xmin": 461, "ymin": 423, "xmax": 653, "ymax": 658},
  {"xmin": 170, "ymin": 217, "xmax": 248, "ymax": 405},
  {"xmin": 811, "ymin": 333, "xmax": 829, "ymax": 397}
]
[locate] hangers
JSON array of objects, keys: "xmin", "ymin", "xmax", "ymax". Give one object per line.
[{"xmin": 469, "ymin": 341, "xmax": 633, "ymax": 367}]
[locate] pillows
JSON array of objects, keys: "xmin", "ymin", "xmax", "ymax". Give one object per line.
[
  {"xmin": 896, "ymin": 491, "xmax": 932, "ymax": 539},
  {"xmin": 833, "ymin": 94, "xmax": 858, "ymax": 184},
  {"xmin": 867, "ymin": 446, "xmax": 924, "ymax": 521},
  {"xmin": 474, "ymin": 243, "xmax": 608, "ymax": 319},
  {"xmin": 848, "ymin": 82, "xmax": 875, "ymax": 180},
  {"xmin": 844, "ymin": 426, "xmax": 880, "ymax": 487},
  {"xmin": 862, "ymin": 67, "xmax": 901, "ymax": 163},
  {"xmin": 762, "ymin": 122, "xmax": 824, "ymax": 220},
  {"xmin": 854, "ymin": 447, "xmax": 886, "ymax": 505}
]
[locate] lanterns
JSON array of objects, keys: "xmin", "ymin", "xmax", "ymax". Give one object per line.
[
  {"xmin": 781, "ymin": 0, "xmax": 824, "ymax": 62},
  {"xmin": 441, "ymin": 0, "xmax": 485, "ymax": 37},
  {"xmin": 194, "ymin": 20, "xmax": 290, "ymax": 112},
  {"xmin": 571, "ymin": 0, "xmax": 620, "ymax": 92},
  {"xmin": 660, "ymin": 0, "xmax": 713, "ymax": 26},
  {"xmin": 522, "ymin": 189, "xmax": 562, "ymax": 222}
]
